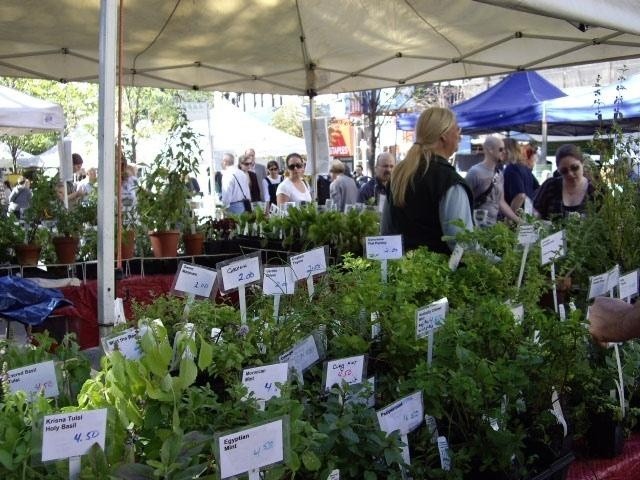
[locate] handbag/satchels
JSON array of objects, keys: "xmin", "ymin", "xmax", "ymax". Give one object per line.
[{"xmin": 244, "ymin": 198, "xmax": 251, "ymax": 210}]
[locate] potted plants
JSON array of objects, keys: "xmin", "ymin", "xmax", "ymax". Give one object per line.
[
  {"xmin": 134, "ymin": 111, "xmax": 184, "ymax": 259},
  {"xmin": 50, "ymin": 204, "xmax": 86, "ymax": 265},
  {"xmin": 0, "ymin": 204, "xmax": 13, "ymax": 268},
  {"xmin": 110, "ymin": 209, "xmax": 135, "ymax": 260},
  {"xmin": 183, "ymin": 222, "xmax": 206, "ymax": 255},
  {"xmin": 212, "ymin": 203, "xmax": 287, "ymax": 267},
  {"xmin": 12, "ymin": 167, "xmax": 55, "ymax": 266}
]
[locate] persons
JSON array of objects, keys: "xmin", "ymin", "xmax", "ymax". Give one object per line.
[
  {"xmin": 383, "ymin": 106, "xmax": 501, "ymax": 267},
  {"xmin": 531, "ymin": 144, "xmax": 604, "ymax": 310},
  {"xmin": 464, "ymin": 136, "xmax": 639, "ymax": 242},
  {"xmin": 1, "ymin": 145, "xmax": 403, "ymax": 242},
  {"xmin": 590, "ymin": 292, "xmax": 640, "ymax": 343}
]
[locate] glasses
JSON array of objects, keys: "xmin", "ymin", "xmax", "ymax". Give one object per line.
[
  {"xmin": 287, "ymin": 163, "xmax": 303, "ymax": 169},
  {"xmin": 244, "ymin": 162, "xmax": 251, "ymax": 165},
  {"xmin": 560, "ymin": 164, "xmax": 578, "ymax": 174},
  {"xmin": 269, "ymin": 168, "xmax": 277, "ymax": 171}
]
